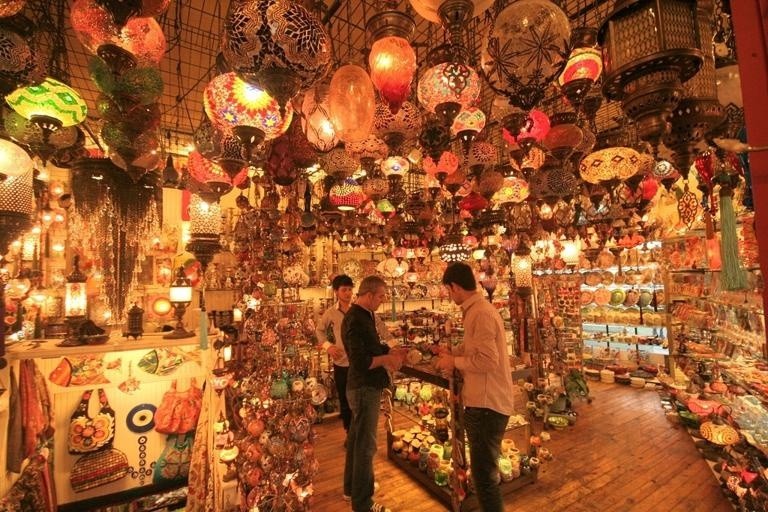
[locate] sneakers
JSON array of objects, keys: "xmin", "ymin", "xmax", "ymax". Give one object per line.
[
  {"xmin": 343, "ymin": 482, "xmax": 380, "ymax": 501},
  {"xmin": 370, "ymin": 501, "xmax": 390, "ymax": 512}
]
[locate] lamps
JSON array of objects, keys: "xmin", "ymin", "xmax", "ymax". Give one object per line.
[{"xmin": 0, "ymin": 0, "xmax": 768, "ymax": 511}]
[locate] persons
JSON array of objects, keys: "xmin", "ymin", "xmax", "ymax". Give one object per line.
[
  {"xmin": 314, "ymin": 275, "xmax": 399, "ymax": 448},
  {"xmin": 340, "ymin": 275, "xmax": 405, "ymax": 512},
  {"xmin": 435, "ymin": 262, "xmax": 517, "ymax": 512}
]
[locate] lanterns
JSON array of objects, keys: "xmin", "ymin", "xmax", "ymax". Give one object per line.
[{"xmin": 1, "ymin": 0, "xmax": 767, "ymax": 353}]
